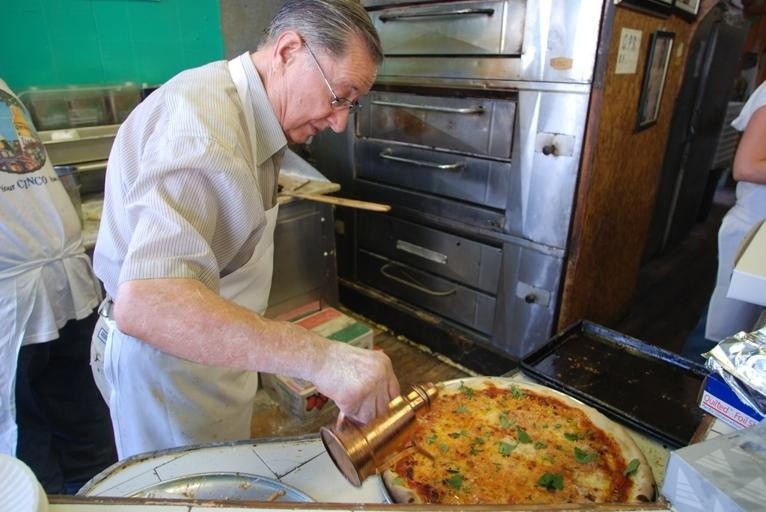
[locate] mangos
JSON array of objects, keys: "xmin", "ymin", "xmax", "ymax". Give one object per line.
[{"xmin": 383, "ymin": 377, "xmax": 654, "ymax": 504}]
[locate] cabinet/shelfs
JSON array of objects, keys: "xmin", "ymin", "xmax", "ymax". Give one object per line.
[{"xmin": 54, "ymin": 163, "xmax": 84, "ymax": 228}]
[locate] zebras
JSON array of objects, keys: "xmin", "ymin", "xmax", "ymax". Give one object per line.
[{"xmin": 328, "ymin": 0, "xmax": 604, "ymax": 358}]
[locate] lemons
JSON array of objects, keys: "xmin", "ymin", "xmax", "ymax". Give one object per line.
[{"xmin": 318, "ymin": 379, "xmax": 435, "ymax": 487}]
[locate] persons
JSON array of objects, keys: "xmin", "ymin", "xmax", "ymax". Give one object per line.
[
  {"xmin": 682, "ymin": 78, "xmax": 766, "ymax": 372},
  {"xmin": 0, "ymin": 78, "xmax": 117, "ymax": 495},
  {"xmin": 88, "ymin": 0, "xmax": 393, "ymax": 462}
]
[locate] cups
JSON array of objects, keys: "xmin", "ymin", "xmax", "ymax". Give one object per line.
[{"xmin": 301, "ymin": 40, "xmax": 365, "ymax": 116}]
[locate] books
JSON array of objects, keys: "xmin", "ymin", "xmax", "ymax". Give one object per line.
[
  {"xmin": 259, "ymin": 306, "xmax": 378, "ymax": 425},
  {"xmin": 23, "ymin": 84, "xmax": 143, "ymax": 132}
]
